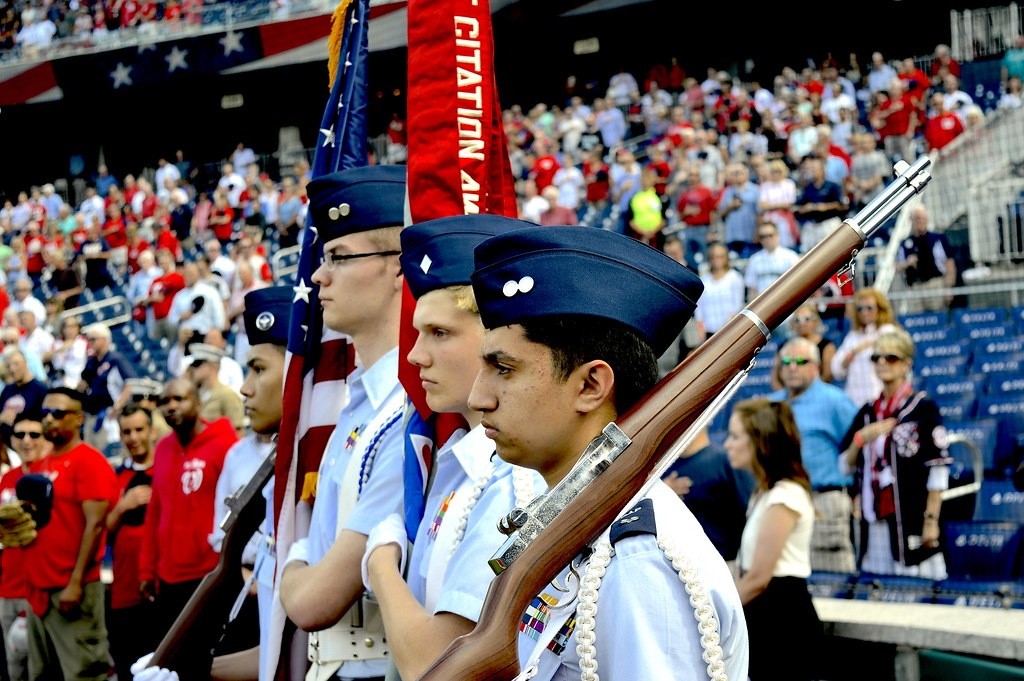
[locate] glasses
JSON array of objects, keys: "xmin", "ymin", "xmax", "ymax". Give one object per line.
[
  {"xmin": 40, "ymin": 409, "xmax": 77, "ymax": 419},
  {"xmin": 872, "ymin": 354, "xmax": 905, "ymax": 364},
  {"xmin": 857, "ymin": 305, "xmax": 874, "ymax": 311},
  {"xmin": 320, "ymin": 250, "xmax": 401, "ymax": 271},
  {"xmin": 133, "ymin": 394, "xmax": 156, "ymax": 402},
  {"xmin": 782, "ymin": 357, "xmax": 808, "ymax": 365},
  {"xmin": 14, "ymin": 432, "xmax": 42, "ymax": 439}
]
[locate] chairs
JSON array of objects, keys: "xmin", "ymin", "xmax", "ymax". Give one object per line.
[{"xmin": 703, "ymin": 301, "xmax": 1023, "ymax": 582}]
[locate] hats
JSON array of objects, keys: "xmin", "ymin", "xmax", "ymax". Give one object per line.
[
  {"xmin": 306, "ymin": 165, "xmax": 406, "ymax": 242},
  {"xmin": 189, "ymin": 344, "xmax": 223, "ymax": 361},
  {"xmin": 400, "ymin": 214, "xmax": 543, "ymax": 300},
  {"xmin": 471, "ymin": 225, "xmax": 704, "ymax": 357},
  {"xmin": 124, "ymin": 378, "xmax": 163, "ymax": 398},
  {"xmin": 15, "ymin": 475, "xmax": 53, "ymax": 509},
  {"xmin": 243, "ymin": 285, "xmax": 295, "ymax": 344}
]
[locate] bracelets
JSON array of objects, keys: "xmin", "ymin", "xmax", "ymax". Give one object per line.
[
  {"xmin": 854, "ymin": 432, "xmax": 865, "ymax": 446},
  {"xmin": 924, "ymin": 511, "xmax": 939, "ymax": 519}
]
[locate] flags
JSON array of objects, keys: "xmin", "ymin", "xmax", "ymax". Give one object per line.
[
  {"xmin": 398, "ymin": 1, "xmax": 518, "ymax": 543},
  {"xmin": 294, "ymin": 0, "xmax": 372, "ymax": 506}
]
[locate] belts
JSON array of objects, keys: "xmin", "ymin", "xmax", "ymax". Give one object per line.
[{"xmin": 307, "ymin": 595, "xmax": 388, "ymax": 662}]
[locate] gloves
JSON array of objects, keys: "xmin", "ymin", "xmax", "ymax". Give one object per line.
[
  {"xmin": 131, "ymin": 652, "xmax": 179, "ymax": 681},
  {"xmin": 361, "ymin": 513, "xmax": 408, "ymax": 592},
  {"xmin": 281, "ymin": 538, "xmax": 308, "ymax": 579}
]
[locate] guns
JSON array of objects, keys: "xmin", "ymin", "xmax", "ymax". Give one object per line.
[
  {"xmin": 145, "ymin": 433, "xmax": 278, "ymax": 681},
  {"xmin": 412, "ymin": 154, "xmax": 932, "ymax": 681}
]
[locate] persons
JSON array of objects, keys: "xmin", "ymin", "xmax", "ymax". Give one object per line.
[
  {"xmin": 0, "ymin": 0, "xmax": 291, "ymax": 59},
  {"xmin": 361, "ymin": 213, "xmax": 539, "ymax": 681},
  {"xmin": 470, "ymin": 226, "xmax": 748, "ymax": 681},
  {"xmin": 128, "ymin": 286, "xmax": 293, "ymax": 681},
  {"xmin": 661, "ymin": 283, "xmax": 950, "ymax": 681},
  {"xmin": 0, "ymin": 137, "xmax": 273, "ymax": 681},
  {"xmin": 237, "ymin": 40, "xmax": 1024, "ymax": 368},
  {"xmin": 275, "ymin": 164, "xmax": 407, "ymax": 681}
]
